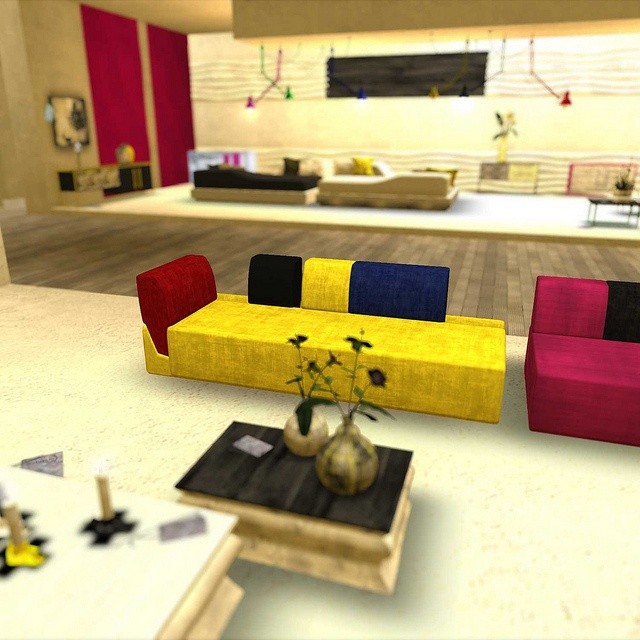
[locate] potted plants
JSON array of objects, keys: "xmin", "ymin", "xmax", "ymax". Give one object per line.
[{"xmin": 609, "ymin": 167, "xmax": 635, "ymax": 195}]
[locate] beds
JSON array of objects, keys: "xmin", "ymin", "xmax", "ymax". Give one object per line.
[{"xmin": 174, "ymin": 422, "xmax": 416, "ymax": 595}]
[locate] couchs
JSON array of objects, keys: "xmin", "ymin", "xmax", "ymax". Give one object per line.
[
  {"xmin": 316, "ymin": 173, "xmax": 459, "ymax": 210},
  {"xmin": 135, "ymin": 254, "xmax": 506, "ymax": 423},
  {"xmin": 191, "ymin": 165, "xmax": 321, "ymax": 206},
  {"xmin": 524, "ymin": 275, "xmax": 640, "ymax": 446}
]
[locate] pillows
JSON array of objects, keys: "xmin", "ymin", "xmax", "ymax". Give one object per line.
[{"xmin": 351, "ymin": 155, "xmax": 375, "ymax": 174}]
